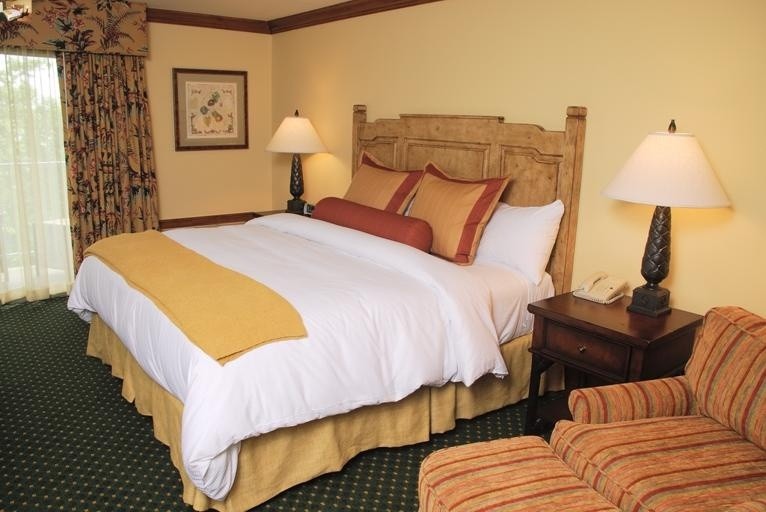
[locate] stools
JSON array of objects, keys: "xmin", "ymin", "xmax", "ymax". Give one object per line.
[{"xmin": 412, "ymin": 432, "xmax": 620, "ymax": 511}]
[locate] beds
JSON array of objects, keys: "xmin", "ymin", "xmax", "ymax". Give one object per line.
[{"xmin": 81, "ymin": 103, "xmax": 587, "ymax": 512}]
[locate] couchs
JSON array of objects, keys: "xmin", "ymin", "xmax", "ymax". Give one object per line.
[{"xmin": 549, "ymin": 305, "xmax": 766, "ymax": 512}]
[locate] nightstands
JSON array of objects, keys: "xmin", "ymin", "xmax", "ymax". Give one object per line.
[{"xmin": 522, "ymin": 292, "xmax": 704, "ymax": 435}]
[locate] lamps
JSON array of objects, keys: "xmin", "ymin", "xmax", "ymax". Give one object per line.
[
  {"xmin": 599, "ymin": 118, "xmax": 731, "ymax": 317},
  {"xmin": 264, "ymin": 109, "xmax": 330, "ymax": 217}
]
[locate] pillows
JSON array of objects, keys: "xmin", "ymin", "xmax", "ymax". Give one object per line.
[{"xmin": 309, "ymin": 150, "xmax": 565, "ymax": 287}]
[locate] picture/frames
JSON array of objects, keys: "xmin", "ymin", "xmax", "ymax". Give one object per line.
[{"xmin": 171, "ymin": 67, "xmax": 248, "ymax": 151}]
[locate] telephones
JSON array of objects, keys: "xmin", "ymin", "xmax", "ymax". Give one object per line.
[{"xmin": 573, "ymin": 271, "xmax": 628, "ymax": 304}]
